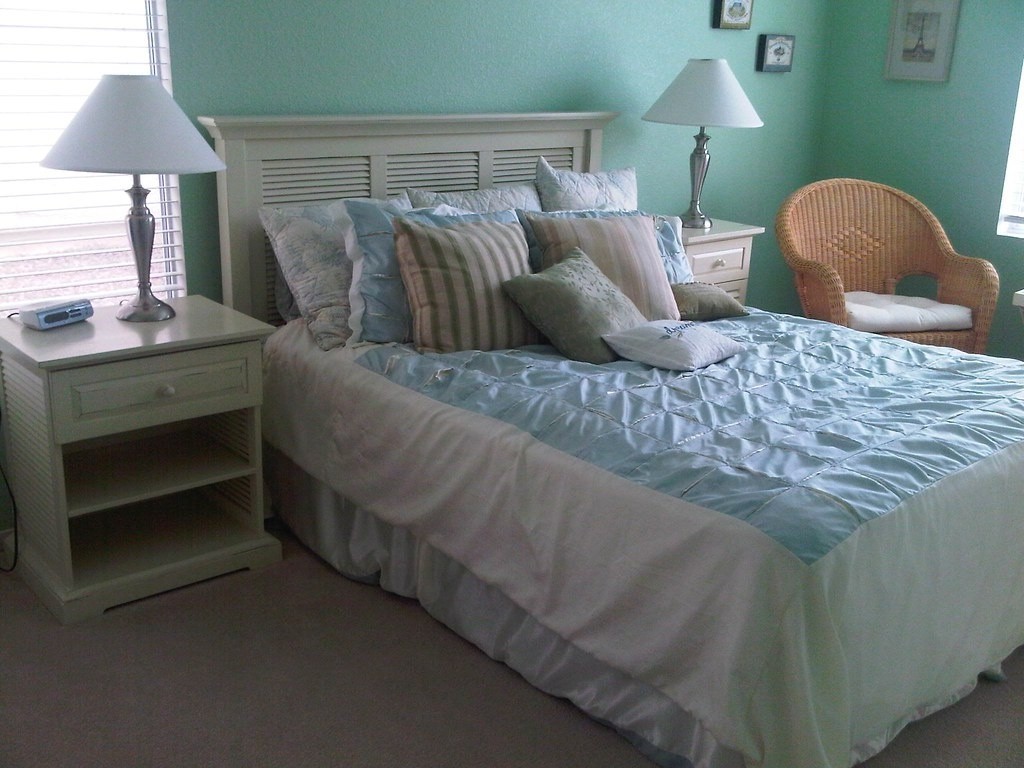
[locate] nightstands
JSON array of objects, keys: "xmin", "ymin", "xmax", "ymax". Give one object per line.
[
  {"xmin": 0, "ymin": 293, "xmax": 286, "ymax": 626},
  {"xmin": 679, "ymin": 218, "xmax": 766, "ymax": 308}
]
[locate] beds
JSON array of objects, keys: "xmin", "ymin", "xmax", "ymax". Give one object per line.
[{"xmin": 198, "ymin": 110, "xmax": 1023, "ymax": 768}]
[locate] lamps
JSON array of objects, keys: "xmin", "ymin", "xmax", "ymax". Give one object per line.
[
  {"xmin": 42, "ymin": 73, "xmax": 227, "ymax": 322},
  {"xmin": 641, "ymin": 58, "xmax": 766, "ymax": 229}
]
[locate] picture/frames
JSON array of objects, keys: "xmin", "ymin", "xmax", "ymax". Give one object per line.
[{"xmin": 884, "ymin": 0, "xmax": 960, "ymax": 82}]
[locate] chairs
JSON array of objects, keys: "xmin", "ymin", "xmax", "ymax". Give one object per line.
[{"xmin": 775, "ymin": 176, "xmax": 1000, "ymax": 355}]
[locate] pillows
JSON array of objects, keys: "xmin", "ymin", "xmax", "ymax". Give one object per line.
[
  {"xmin": 671, "ymin": 281, "xmax": 749, "ymax": 322},
  {"xmin": 257, "ymin": 192, "xmax": 416, "ymax": 349},
  {"xmin": 844, "ymin": 288, "xmax": 974, "ymax": 333},
  {"xmin": 534, "ymin": 157, "xmax": 640, "ymax": 211},
  {"xmin": 329, "ymin": 199, "xmax": 546, "ymax": 351},
  {"xmin": 391, "ymin": 215, "xmax": 550, "ymax": 352},
  {"xmin": 602, "ymin": 319, "xmax": 748, "ymax": 369},
  {"xmin": 405, "ymin": 182, "xmax": 543, "ymax": 214},
  {"xmin": 525, "ymin": 211, "xmax": 682, "ymax": 322},
  {"xmin": 516, "ymin": 207, "xmax": 694, "ymax": 284},
  {"xmin": 501, "ymin": 245, "xmax": 649, "ymax": 365}
]
